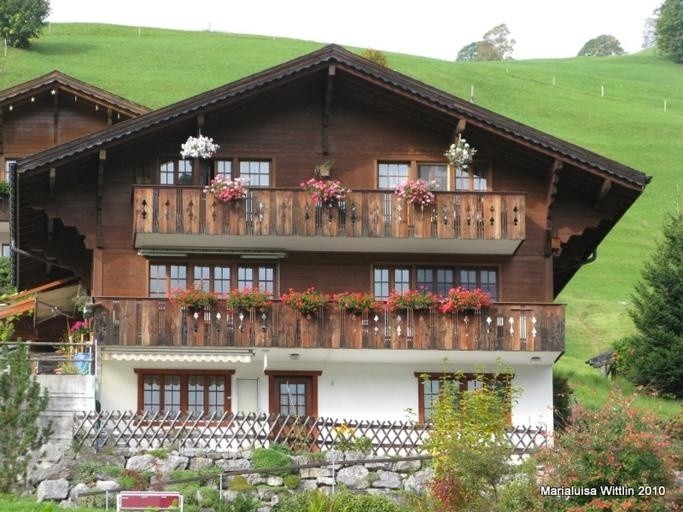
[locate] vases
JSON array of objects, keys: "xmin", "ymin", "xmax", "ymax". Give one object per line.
[{"xmin": 73, "ymin": 353, "xmax": 89, "ymax": 375}]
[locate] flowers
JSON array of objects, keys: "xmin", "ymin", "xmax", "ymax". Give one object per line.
[
  {"xmin": 394, "ymin": 178, "xmax": 440, "ymax": 207},
  {"xmin": 203, "ymin": 174, "xmax": 246, "ymax": 202},
  {"xmin": 442, "ymin": 133, "xmax": 477, "ymax": 169},
  {"xmin": 180, "ymin": 134, "xmax": 221, "ymax": 161},
  {"xmin": 166, "ymin": 285, "xmax": 494, "ymax": 316},
  {"xmin": 71, "ymin": 321, "xmax": 91, "ymax": 353},
  {"xmin": 300, "ymin": 178, "xmax": 352, "ymax": 209}
]
